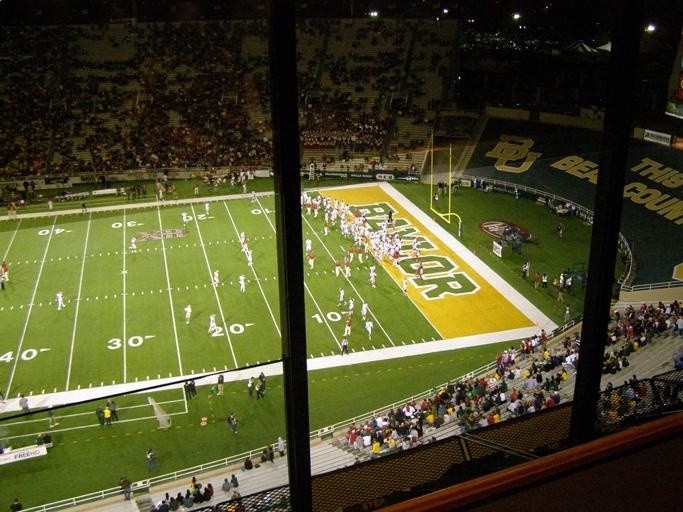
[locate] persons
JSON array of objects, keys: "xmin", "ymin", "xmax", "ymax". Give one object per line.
[
  {"xmin": 9, "ymin": 497, "xmax": 22, "ymax": 512},
  {"xmin": 346, "ymin": 300, "xmax": 682, "ymax": 461},
  {"xmin": 146, "ymin": 448, "xmax": 156, "ymax": 463},
  {"xmin": 519, "ymin": 259, "xmax": 572, "ymax": 302},
  {"xmin": 307, "ymin": 220, "xmax": 426, "ymax": 294},
  {"xmin": 159, "ymin": 436, "xmax": 293, "ymax": 511},
  {"xmin": 213, "ymin": 232, "xmax": 254, "ymax": 293},
  {"xmin": 248, "ymin": 373, "xmax": 267, "ymax": 399},
  {"xmin": 184, "ymin": 379, "xmax": 197, "ymax": 400},
  {"xmin": 19, "ymin": 395, "xmax": 30, "ymax": 416},
  {"xmin": 95, "ymin": 400, "xmax": 118, "ymax": 425},
  {"xmin": 119, "ymin": 476, "xmax": 131, "ymax": 500},
  {"xmin": 56, "ymin": 289, "xmax": 67, "ymax": 311},
  {"xmin": 0, "ymin": 0, "xmax": 461, "ymax": 220},
  {"xmin": 129, "ymin": 236, "xmax": 137, "ymax": 252},
  {"xmin": 341, "ymin": 298, "xmax": 373, "ymax": 356},
  {"xmin": 46, "ymin": 404, "xmax": 59, "ymax": 427},
  {"xmin": 218, "ymin": 375, "xmax": 224, "ymax": 396},
  {"xmin": 229, "ymin": 412, "xmax": 239, "ymax": 426},
  {"xmin": 1, "ymin": 260, "xmax": 10, "ymax": 290}
]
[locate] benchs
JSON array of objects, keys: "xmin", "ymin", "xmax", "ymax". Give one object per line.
[
  {"xmin": 0, "ymin": 17, "xmax": 456, "ymax": 174},
  {"xmin": 94, "ymin": 325, "xmax": 682, "ymax": 512}
]
